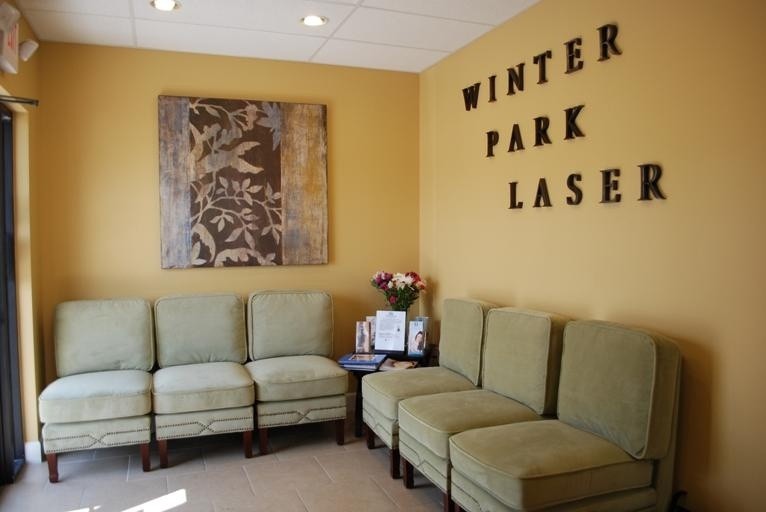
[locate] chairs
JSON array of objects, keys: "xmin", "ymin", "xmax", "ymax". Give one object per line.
[
  {"xmin": 36, "ymin": 296, "xmax": 155, "ymax": 486},
  {"xmin": 151, "ymin": 292, "xmax": 256, "ymax": 471},
  {"xmin": 446, "ymin": 317, "xmax": 685, "ymax": 512},
  {"xmin": 245, "ymin": 288, "xmax": 350, "ymax": 456},
  {"xmin": 396, "ymin": 305, "xmax": 561, "ymax": 511},
  {"xmin": 359, "ymin": 297, "xmax": 484, "ymax": 481}
]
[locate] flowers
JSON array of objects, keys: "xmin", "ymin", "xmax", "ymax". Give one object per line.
[{"xmin": 369, "ymin": 267, "xmax": 428, "ymax": 314}]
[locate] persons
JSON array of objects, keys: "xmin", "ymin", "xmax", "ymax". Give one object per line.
[
  {"xmin": 389, "ymin": 360, "xmax": 415, "ymax": 368},
  {"xmin": 410, "ymin": 330, "xmax": 423, "ymax": 351}
]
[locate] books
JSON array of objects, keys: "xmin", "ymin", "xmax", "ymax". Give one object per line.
[
  {"xmin": 380, "ymin": 358, "xmax": 419, "ymax": 371},
  {"xmin": 343, "ymin": 362, "xmax": 380, "ymax": 369}
]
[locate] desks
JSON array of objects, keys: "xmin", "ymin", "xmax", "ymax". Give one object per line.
[{"xmin": 351, "ymin": 345, "xmax": 434, "ymax": 439}]
